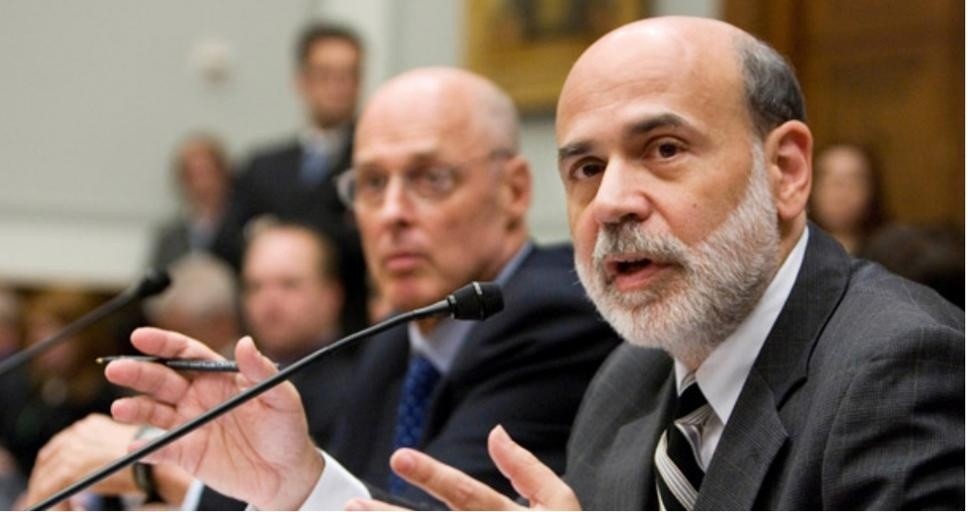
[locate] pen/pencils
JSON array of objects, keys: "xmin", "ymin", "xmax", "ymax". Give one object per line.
[{"xmin": 95, "ymin": 355, "xmax": 285, "ymax": 372}]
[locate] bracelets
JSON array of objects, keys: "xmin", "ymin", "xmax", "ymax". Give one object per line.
[{"xmin": 129, "ymin": 421, "xmax": 168, "ymax": 502}]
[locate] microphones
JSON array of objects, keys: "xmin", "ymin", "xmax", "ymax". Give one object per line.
[
  {"xmin": 0, "ymin": 267, "xmax": 172, "ymax": 377},
  {"xmin": 24, "ymin": 281, "xmax": 504, "ymax": 510}
]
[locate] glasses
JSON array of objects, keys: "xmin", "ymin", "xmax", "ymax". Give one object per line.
[{"xmin": 331, "ymin": 145, "xmax": 512, "ymax": 217}]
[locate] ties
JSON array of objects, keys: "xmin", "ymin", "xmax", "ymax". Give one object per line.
[
  {"xmin": 385, "ymin": 350, "xmax": 440, "ymax": 494},
  {"xmin": 651, "ymin": 370, "xmax": 716, "ymax": 510}
]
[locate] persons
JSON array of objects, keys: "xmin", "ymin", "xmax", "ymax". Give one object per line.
[
  {"xmin": 104, "ymin": 15, "xmax": 964, "ymax": 511},
  {"xmin": 235, "ymin": 213, "xmax": 348, "ymax": 371},
  {"xmin": 25, "ymin": 63, "xmax": 624, "ymax": 511},
  {"xmin": 220, "ymin": 23, "xmax": 365, "ymax": 329},
  {"xmin": 808, "ymin": 139, "xmax": 888, "ymax": 255}
]
